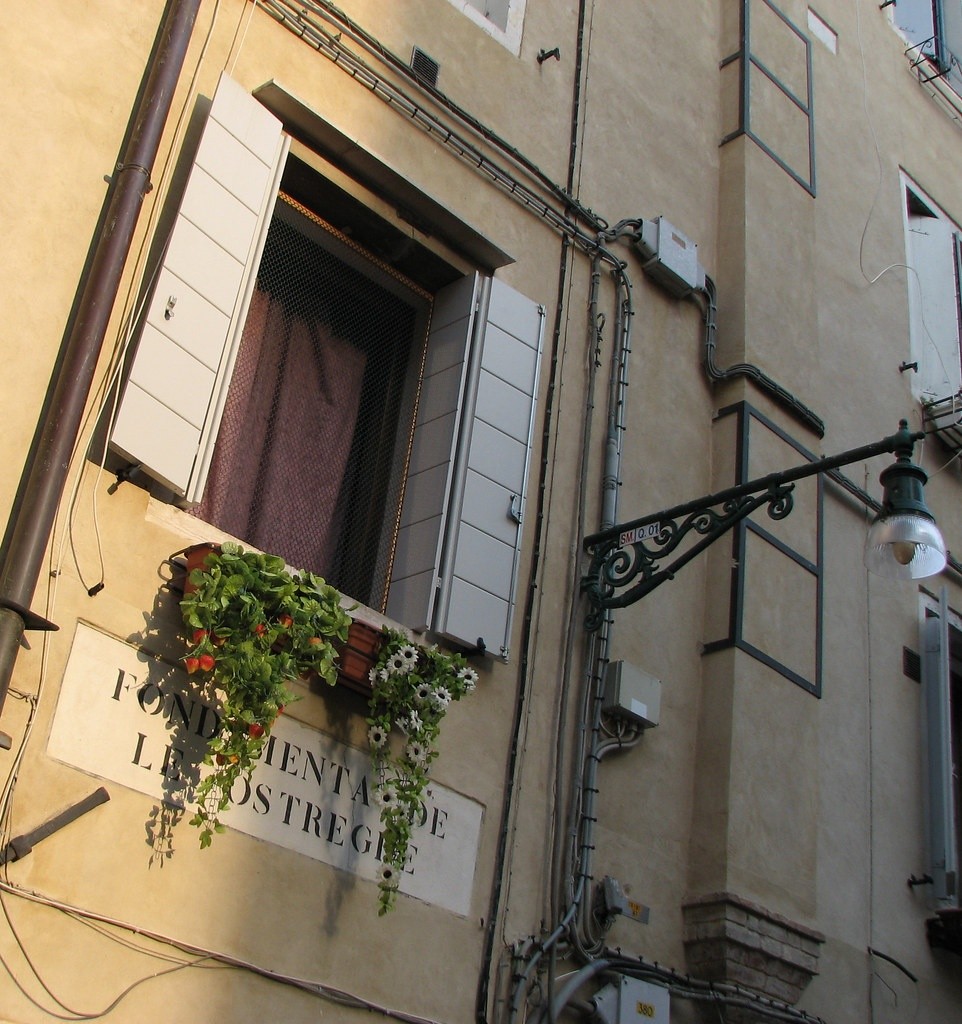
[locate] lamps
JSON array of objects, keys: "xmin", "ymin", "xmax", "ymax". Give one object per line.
[{"xmin": 576, "ymin": 418, "xmax": 948, "ymax": 633}]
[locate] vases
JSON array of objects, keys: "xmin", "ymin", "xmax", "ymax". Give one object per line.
[{"xmin": 182, "ymin": 541, "xmax": 443, "ymax": 699}]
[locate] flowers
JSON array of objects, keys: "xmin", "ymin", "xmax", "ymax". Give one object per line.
[{"xmin": 157, "ymin": 545, "xmax": 481, "ymax": 916}]
